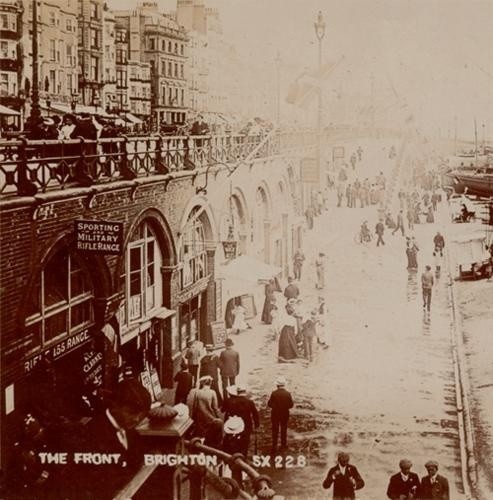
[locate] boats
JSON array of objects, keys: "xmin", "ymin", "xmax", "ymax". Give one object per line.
[{"xmin": 442, "ymin": 113, "xmax": 493, "ymax": 282}]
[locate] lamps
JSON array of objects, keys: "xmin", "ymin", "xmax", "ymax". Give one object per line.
[{"xmin": 194, "ymin": 162, "xmax": 238, "ymax": 261}]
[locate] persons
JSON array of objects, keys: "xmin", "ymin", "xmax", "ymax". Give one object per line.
[
  {"xmin": 0, "ymin": 103, "xmax": 454, "ymax": 500},
  {"xmin": 456, "ymin": 203, "xmax": 469, "ymax": 220}
]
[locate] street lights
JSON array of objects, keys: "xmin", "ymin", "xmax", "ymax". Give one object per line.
[
  {"xmin": 111, "ymin": 99, "xmax": 117, "ymax": 124},
  {"xmin": 91, "ymin": 93, "xmax": 101, "ymax": 114},
  {"xmin": 70, "ymin": 92, "xmax": 80, "ymax": 114},
  {"xmin": 313, "ymin": 10, "xmax": 328, "ymax": 140},
  {"xmin": 123, "ymin": 101, "xmax": 129, "ymax": 129},
  {"xmin": 45, "ymin": 96, "xmax": 52, "ymax": 117},
  {"xmin": 271, "ymin": 50, "xmax": 282, "ymax": 130}
]
